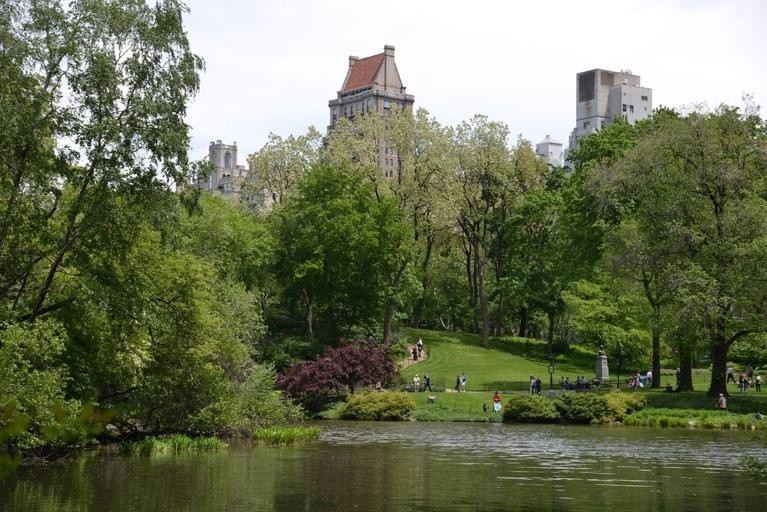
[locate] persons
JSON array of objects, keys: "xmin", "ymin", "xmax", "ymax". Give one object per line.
[
  {"xmin": 675, "ymin": 367, "xmax": 680, "ymax": 386},
  {"xmin": 754, "ymin": 373, "xmax": 760, "ymax": 392},
  {"xmin": 738, "ymin": 372, "xmax": 745, "ymax": 392},
  {"xmin": 455, "ymin": 375, "xmax": 460, "ymax": 392},
  {"xmin": 725, "ymin": 365, "xmax": 735, "ymax": 383},
  {"xmin": 746, "ymin": 366, "xmax": 753, "ymax": 382},
  {"xmin": 528, "ymin": 370, "xmax": 652, "ymax": 395},
  {"xmin": 421, "ymin": 374, "xmax": 431, "ymax": 392},
  {"xmin": 412, "ymin": 374, "xmax": 419, "ymax": 392},
  {"xmin": 459, "ymin": 372, "xmax": 467, "ymax": 392},
  {"xmin": 491, "ymin": 391, "xmax": 501, "ymax": 413},
  {"xmin": 713, "ymin": 393, "xmax": 726, "ymax": 411}
]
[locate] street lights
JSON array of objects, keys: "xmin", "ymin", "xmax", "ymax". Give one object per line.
[{"xmin": 548, "ymin": 350, "xmax": 554, "ymax": 390}]
